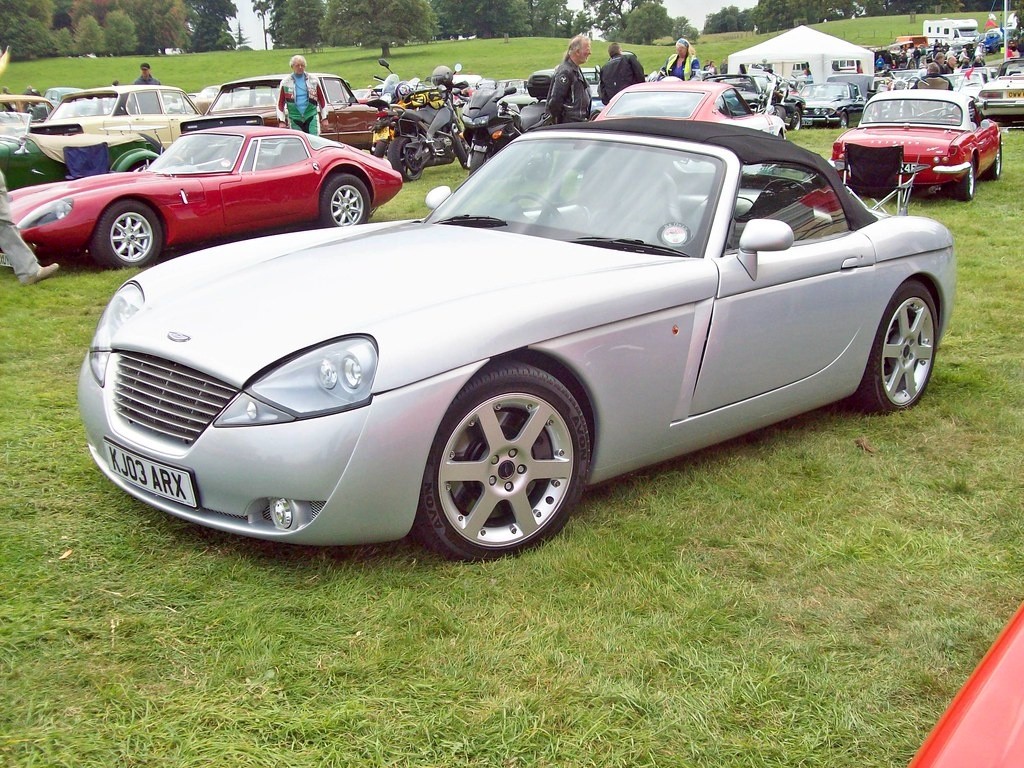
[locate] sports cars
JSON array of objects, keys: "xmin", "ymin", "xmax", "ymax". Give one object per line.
[{"xmin": 7, "ymin": 126, "xmax": 403, "ymax": 270}]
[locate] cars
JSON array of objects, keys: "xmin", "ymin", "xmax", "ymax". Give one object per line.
[
  {"xmin": 829, "ymin": 88, "xmax": 1003, "ymax": 202},
  {"xmin": 976, "ymin": 57, "xmax": 1024, "ymax": 128},
  {"xmin": 580, "ymin": 66, "xmax": 998, "ymax": 129},
  {"xmin": 0, "ymin": 73, "xmax": 380, "ymax": 153},
  {"xmin": 77, "ymin": 116, "xmax": 955, "ymax": 563}
]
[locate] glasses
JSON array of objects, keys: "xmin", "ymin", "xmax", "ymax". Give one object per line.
[{"xmin": 141, "ymin": 68, "xmax": 149, "ymax": 71}]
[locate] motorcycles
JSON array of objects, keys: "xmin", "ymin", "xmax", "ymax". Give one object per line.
[{"xmin": 372, "ymin": 59, "xmax": 557, "ymax": 184}]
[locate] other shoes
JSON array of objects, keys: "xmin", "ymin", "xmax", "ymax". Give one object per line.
[{"xmin": 22, "ymin": 263, "xmax": 59, "ymax": 285}]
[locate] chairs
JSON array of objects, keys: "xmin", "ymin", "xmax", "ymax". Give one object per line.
[
  {"xmin": 590, "ymin": 164, "xmax": 683, "ymax": 245},
  {"xmin": 917, "ymin": 77, "xmax": 949, "ymax": 90},
  {"xmin": 259, "ymin": 96, "xmax": 275, "ymax": 105},
  {"xmin": 63, "ymin": 142, "xmax": 112, "ymax": 181},
  {"xmin": 836, "ymin": 141, "xmax": 930, "ymax": 217},
  {"xmin": 1009, "ymin": 70, "xmax": 1021, "ymax": 76}
]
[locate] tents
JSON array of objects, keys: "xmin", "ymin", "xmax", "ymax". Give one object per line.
[{"xmin": 728, "ymin": 25, "xmax": 874, "ymax": 83}]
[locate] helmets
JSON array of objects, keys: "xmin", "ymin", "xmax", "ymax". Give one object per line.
[
  {"xmin": 432, "ymin": 66, "xmax": 453, "ymax": 86},
  {"xmin": 394, "ymin": 81, "xmax": 414, "ymax": 101}
]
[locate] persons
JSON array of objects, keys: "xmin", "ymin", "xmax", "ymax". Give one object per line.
[
  {"xmin": 599, "ymin": 42, "xmax": 645, "ymax": 105},
  {"xmin": 2, "ymin": 87, "xmax": 16, "ymax": 111},
  {"xmin": 0, "ymin": 172, "xmax": 59, "ymax": 286},
  {"xmin": 971, "ymin": 43, "xmax": 986, "ymax": 67},
  {"xmin": 132, "ymin": 63, "xmax": 160, "ymax": 85},
  {"xmin": 857, "ymin": 61, "xmax": 863, "ymax": 72},
  {"xmin": 738, "ymin": 64, "xmax": 750, "ymax": 82},
  {"xmin": 804, "ymin": 63, "xmax": 813, "ymax": 83},
  {"xmin": 660, "ymin": 38, "xmax": 699, "ymax": 81},
  {"xmin": 876, "ymin": 38, "xmax": 971, "ymax": 91},
  {"xmin": 1016, "ymin": 39, "xmax": 1024, "ymax": 57},
  {"xmin": 276, "ymin": 55, "xmax": 329, "ymax": 136},
  {"xmin": 703, "ymin": 60, "xmax": 717, "ymax": 76},
  {"xmin": 543, "ymin": 35, "xmax": 591, "ymax": 124},
  {"xmin": 22, "ymin": 86, "xmax": 38, "ymax": 111},
  {"xmin": 720, "ymin": 58, "xmax": 728, "ymax": 74}
]
